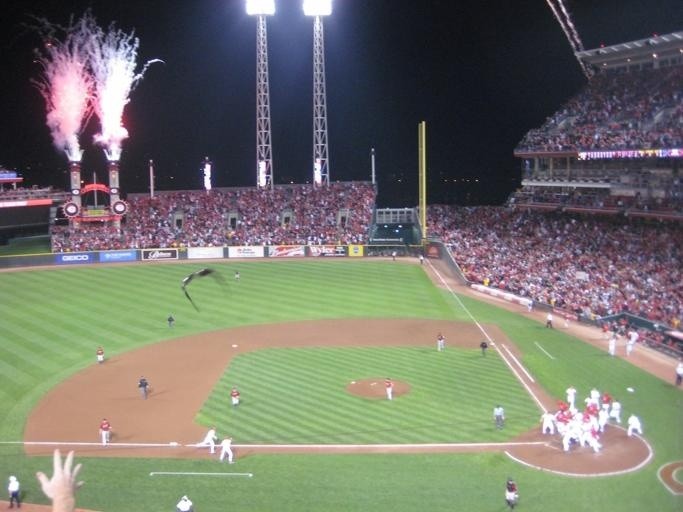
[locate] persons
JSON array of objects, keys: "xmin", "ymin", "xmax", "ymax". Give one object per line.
[
  {"xmin": 503, "ymin": 478, "xmax": 518, "ymax": 510},
  {"xmin": 138, "ymin": 375, "xmax": 149, "ymax": 400},
  {"xmin": 167, "ymin": 316, "xmax": 174, "ymax": 328},
  {"xmin": 415, "ymin": 65, "xmax": 682, "ymax": 359},
  {"xmin": 384, "ymin": 378, "xmax": 392, "ymax": 400},
  {"xmin": 538, "ymin": 386, "xmax": 643, "ymax": 455},
  {"xmin": 176, "ymin": 497, "xmax": 193, "ymax": 512},
  {"xmin": 230, "ymin": 387, "xmax": 240, "ymax": 405},
  {"xmin": 391, "ymin": 249, "xmax": 396, "ymax": 260},
  {"xmin": 99, "ymin": 418, "xmax": 111, "ymax": 447},
  {"xmin": 675, "ymin": 359, "xmax": 682, "ymax": 387},
  {"xmin": 7, "ymin": 475, "xmax": 20, "ymax": 508},
  {"xmin": 234, "ymin": 269, "xmax": 240, "ymax": 281},
  {"xmin": 219, "ymin": 436, "xmax": 236, "ymax": 464},
  {"xmin": 493, "ymin": 404, "xmax": 505, "ymax": 431},
  {"xmin": 195, "ymin": 425, "xmax": 218, "ymax": 454},
  {"xmin": 0, "ymin": 181, "xmax": 376, "ymax": 253},
  {"xmin": 35, "ymin": 448, "xmax": 86, "ymax": 512},
  {"xmin": 96, "ymin": 346, "xmax": 105, "ymax": 363}
]
